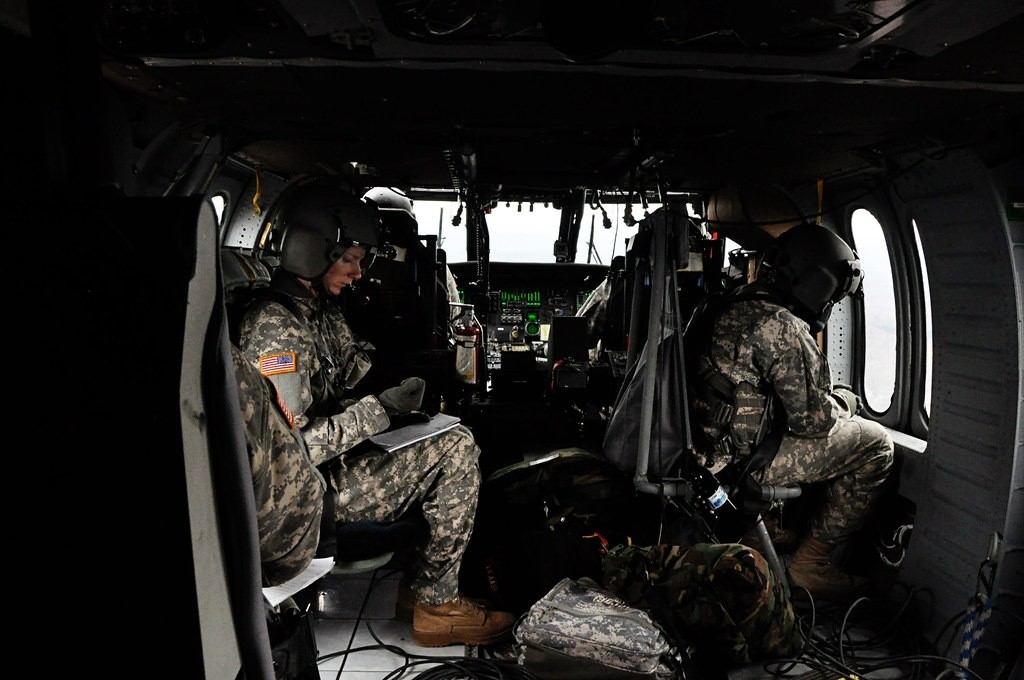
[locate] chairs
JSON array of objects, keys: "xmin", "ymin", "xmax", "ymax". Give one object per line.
[
  {"xmin": 0, "ymin": 193, "xmax": 427, "ymax": 679},
  {"xmin": 624, "ymin": 231, "xmax": 802, "ymax": 601}
]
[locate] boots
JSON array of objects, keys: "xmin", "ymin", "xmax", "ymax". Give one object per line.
[
  {"xmin": 786, "ymin": 533, "xmax": 864, "ymax": 594},
  {"xmin": 744, "ymin": 511, "xmax": 795, "ymax": 551}
]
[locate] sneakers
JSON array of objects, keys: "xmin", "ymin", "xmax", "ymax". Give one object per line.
[
  {"xmin": 393, "ymin": 577, "xmax": 417, "ymax": 621},
  {"xmin": 413, "ymin": 592, "xmax": 515, "ymax": 647}
]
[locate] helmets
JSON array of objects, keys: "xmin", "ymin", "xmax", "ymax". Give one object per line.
[
  {"xmin": 364, "ymin": 187, "xmax": 417, "ymax": 222},
  {"xmin": 764, "ymin": 223, "xmax": 857, "ymax": 314},
  {"xmin": 272, "ymin": 180, "xmax": 379, "ymax": 280}
]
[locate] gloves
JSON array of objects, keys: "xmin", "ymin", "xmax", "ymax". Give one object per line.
[{"xmin": 377, "ymin": 377, "xmax": 425, "ymax": 417}]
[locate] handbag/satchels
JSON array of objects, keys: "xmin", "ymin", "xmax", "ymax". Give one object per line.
[{"xmin": 515, "ymin": 575, "xmax": 694, "ymax": 680}]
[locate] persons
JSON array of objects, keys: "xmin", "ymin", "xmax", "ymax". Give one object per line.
[
  {"xmin": 230, "ymin": 187, "xmax": 517, "ymax": 647},
  {"xmin": 692, "ymin": 223, "xmax": 894, "ymax": 600}
]
